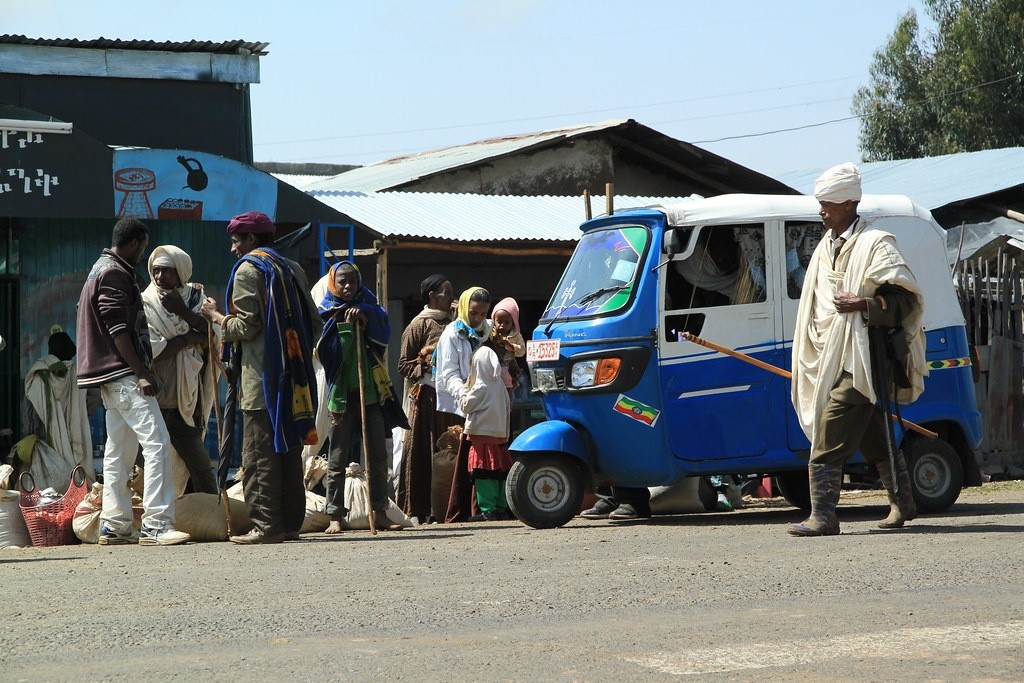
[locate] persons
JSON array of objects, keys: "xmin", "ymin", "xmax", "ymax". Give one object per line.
[
  {"xmin": 138, "ymin": 244, "xmax": 220, "ymax": 496},
  {"xmin": 397, "ymin": 275, "xmax": 527, "ymax": 524},
  {"xmin": 579, "ymin": 485, "xmax": 652, "ymax": 520},
  {"xmin": 316, "ymin": 261, "xmax": 405, "ymax": 534},
  {"xmin": 787, "ymin": 161, "xmax": 929, "ymax": 535},
  {"xmin": 199, "ymin": 213, "xmax": 322, "ymax": 544},
  {"xmin": 75, "ymin": 217, "xmax": 190, "ymax": 546},
  {"xmin": 22, "ymin": 333, "xmax": 96, "ymax": 482}
]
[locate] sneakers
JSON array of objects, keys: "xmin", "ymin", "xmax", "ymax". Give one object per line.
[
  {"xmin": 139, "ymin": 523, "xmax": 190, "ymax": 546},
  {"xmin": 580, "ymin": 506, "xmax": 610, "ymax": 518},
  {"xmin": 610, "ymin": 507, "xmax": 639, "ymax": 519},
  {"xmin": 99, "ymin": 525, "xmax": 141, "ymax": 544},
  {"xmin": 230, "ymin": 528, "xmax": 283, "ymax": 543},
  {"xmin": 283, "ymin": 530, "xmax": 299, "ymax": 540}
]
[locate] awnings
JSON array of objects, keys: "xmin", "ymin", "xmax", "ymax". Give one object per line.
[{"xmin": 311, "ymin": 195, "xmax": 706, "ymax": 240}]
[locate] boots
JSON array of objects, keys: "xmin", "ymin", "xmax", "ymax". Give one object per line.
[
  {"xmin": 875, "ymin": 450, "xmax": 918, "ymax": 528},
  {"xmin": 788, "ymin": 464, "xmax": 843, "ymax": 534}
]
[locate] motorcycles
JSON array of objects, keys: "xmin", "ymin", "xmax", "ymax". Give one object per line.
[{"xmin": 505, "ymin": 191, "xmax": 983, "ymax": 530}]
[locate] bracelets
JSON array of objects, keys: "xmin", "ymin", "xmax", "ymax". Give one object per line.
[
  {"xmin": 180, "ymin": 336, "xmax": 188, "ymax": 347},
  {"xmin": 136, "ymin": 371, "xmax": 148, "ymax": 376}
]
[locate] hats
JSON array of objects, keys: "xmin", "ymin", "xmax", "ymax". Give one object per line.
[
  {"xmin": 227, "ymin": 211, "xmax": 275, "ymax": 233},
  {"xmin": 152, "ymin": 252, "xmax": 176, "ymax": 268},
  {"xmin": 814, "ymin": 162, "xmax": 862, "ymax": 203}
]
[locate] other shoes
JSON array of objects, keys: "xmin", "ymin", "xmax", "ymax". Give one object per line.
[
  {"xmin": 468, "ymin": 511, "xmax": 498, "ymax": 520},
  {"xmin": 494, "ymin": 513, "xmax": 509, "ymax": 519}
]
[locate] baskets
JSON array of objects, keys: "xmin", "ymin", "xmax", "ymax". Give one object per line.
[{"xmin": 19, "ymin": 466, "xmax": 88, "ymax": 547}]
